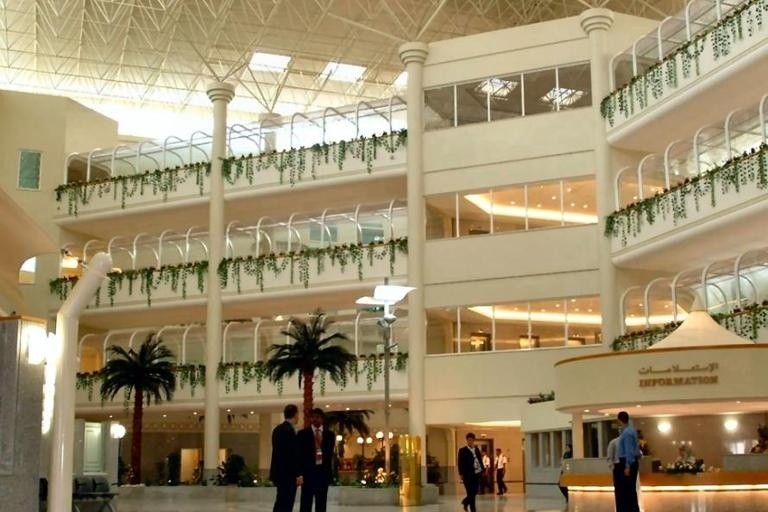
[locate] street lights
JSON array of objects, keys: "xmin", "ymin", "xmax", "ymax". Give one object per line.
[{"xmin": 356, "ymin": 283, "xmax": 416, "ymax": 483}]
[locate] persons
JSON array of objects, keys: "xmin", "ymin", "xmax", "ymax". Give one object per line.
[
  {"xmin": 493, "ymin": 448, "xmax": 508, "ymax": 495},
  {"xmin": 557, "ymin": 444, "xmax": 572, "ymax": 503},
  {"xmin": 295, "ymin": 407, "xmax": 336, "ymax": 511},
  {"xmin": 458, "ymin": 432, "xmax": 484, "ymax": 512},
  {"xmin": 479, "ymin": 448, "xmax": 494, "ymax": 495},
  {"xmin": 269, "ymin": 404, "xmax": 305, "ymax": 511},
  {"xmin": 616, "ymin": 410, "xmax": 641, "ymax": 512},
  {"xmin": 606, "ymin": 425, "xmax": 644, "ymax": 511}
]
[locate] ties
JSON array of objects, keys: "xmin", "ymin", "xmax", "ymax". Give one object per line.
[{"xmin": 315, "ymin": 429, "xmax": 323, "ymax": 465}]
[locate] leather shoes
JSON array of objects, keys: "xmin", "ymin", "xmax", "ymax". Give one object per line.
[{"xmin": 462, "ymin": 499, "xmax": 469, "ymax": 512}]
